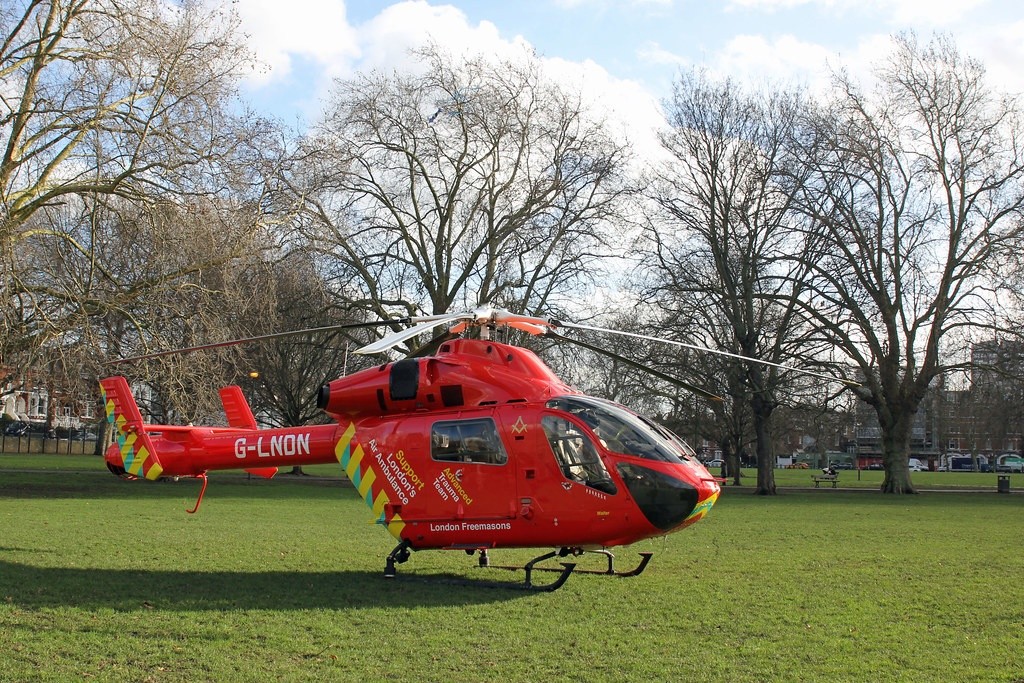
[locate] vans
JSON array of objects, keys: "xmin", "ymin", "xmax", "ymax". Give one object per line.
[{"xmin": 909, "ymin": 458, "xmax": 929, "ymax": 471}]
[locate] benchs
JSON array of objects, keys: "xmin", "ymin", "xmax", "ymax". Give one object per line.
[
  {"xmin": 811, "ymin": 475, "xmax": 835, "ymax": 481},
  {"xmin": 813, "ymin": 480, "xmax": 840, "ymax": 488}
]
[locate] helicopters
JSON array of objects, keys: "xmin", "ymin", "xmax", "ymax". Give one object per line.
[{"xmin": 98, "ymin": 305, "xmax": 862, "ymax": 593}]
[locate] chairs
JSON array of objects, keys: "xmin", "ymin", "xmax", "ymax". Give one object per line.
[{"xmin": 554, "ymin": 437, "xmax": 587, "ymax": 484}]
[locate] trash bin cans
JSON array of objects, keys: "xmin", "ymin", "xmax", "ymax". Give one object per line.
[{"xmin": 997, "ymin": 475, "xmax": 1010, "ymax": 493}]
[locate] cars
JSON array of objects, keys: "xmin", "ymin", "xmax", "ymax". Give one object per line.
[
  {"xmin": 831, "ymin": 462, "xmax": 852, "ymax": 470},
  {"xmin": 909, "ymin": 465, "xmax": 921, "ymax": 471},
  {"xmin": 702, "ymin": 459, "xmax": 724, "ymax": 467},
  {"xmin": 791, "ymin": 462, "xmax": 808, "ymax": 468}
]
